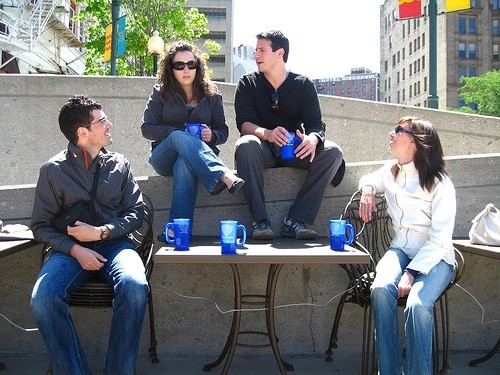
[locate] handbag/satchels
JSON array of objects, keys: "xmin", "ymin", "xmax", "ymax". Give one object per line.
[
  {"xmin": 54, "ymin": 198, "xmax": 107, "ymax": 248},
  {"xmin": 468, "ymin": 203, "xmax": 500, "ymax": 247}
]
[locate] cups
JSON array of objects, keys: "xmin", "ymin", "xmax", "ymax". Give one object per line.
[
  {"xmin": 186, "ymin": 124, "xmax": 207, "ymax": 142},
  {"xmin": 281, "ymin": 131, "xmax": 302, "ymax": 159},
  {"xmin": 163, "ymin": 218, "xmax": 191, "ymax": 252},
  {"xmin": 219, "ymin": 220, "xmax": 246, "ymax": 254},
  {"xmin": 329, "ymin": 219, "xmax": 354, "ymax": 250}
]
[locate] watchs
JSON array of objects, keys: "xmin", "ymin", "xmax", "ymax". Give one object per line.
[{"xmin": 99, "ymin": 227, "xmax": 108, "ymax": 241}]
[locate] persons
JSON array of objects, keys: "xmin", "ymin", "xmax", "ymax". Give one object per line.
[
  {"xmin": 234, "ymin": 30, "xmax": 344, "ymax": 240},
  {"xmin": 358, "ymin": 116, "xmax": 457, "ymax": 375},
  {"xmin": 31, "ymin": 95, "xmax": 150, "ymax": 375},
  {"xmin": 141, "ymin": 42, "xmax": 246, "ymax": 244}
]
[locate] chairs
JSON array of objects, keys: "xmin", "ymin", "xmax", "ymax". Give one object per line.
[
  {"xmin": 325, "ymin": 191, "xmax": 454, "ymax": 375},
  {"xmin": 39, "ymin": 191, "xmax": 160, "ymax": 375}
]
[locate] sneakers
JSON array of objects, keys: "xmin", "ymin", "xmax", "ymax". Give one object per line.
[
  {"xmin": 281, "ymin": 216, "xmax": 317, "ymax": 240},
  {"xmin": 251, "ymin": 221, "xmax": 274, "ymax": 240}
]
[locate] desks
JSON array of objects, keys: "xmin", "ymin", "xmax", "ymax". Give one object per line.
[
  {"xmin": 450, "ymin": 239, "xmax": 500, "ymax": 367},
  {"xmin": 153, "ymin": 235, "xmax": 370, "ymax": 375},
  {"xmin": 0, "ymin": 225, "xmax": 40, "ymax": 258}
]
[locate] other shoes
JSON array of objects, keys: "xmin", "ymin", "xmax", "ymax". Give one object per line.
[
  {"xmin": 226, "ymin": 178, "xmax": 245, "ymax": 195},
  {"xmin": 157, "ymin": 232, "xmax": 175, "ymax": 243}
]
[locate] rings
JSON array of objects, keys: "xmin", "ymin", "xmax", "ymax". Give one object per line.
[{"xmin": 203, "ymin": 136, "xmax": 206, "ymax": 138}]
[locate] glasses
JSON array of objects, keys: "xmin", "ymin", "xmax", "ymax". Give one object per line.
[
  {"xmin": 85, "ymin": 117, "xmax": 107, "ymax": 129},
  {"xmin": 171, "ymin": 60, "xmax": 199, "ymax": 71},
  {"xmin": 394, "ymin": 125, "xmax": 412, "ymax": 136}
]
